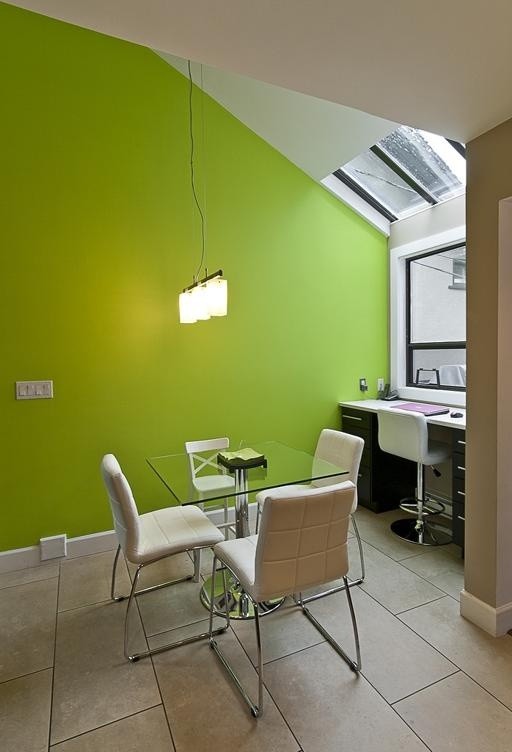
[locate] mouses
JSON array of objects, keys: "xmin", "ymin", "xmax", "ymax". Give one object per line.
[{"xmin": 451, "ymin": 412, "xmax": 463, "ymax": 417}]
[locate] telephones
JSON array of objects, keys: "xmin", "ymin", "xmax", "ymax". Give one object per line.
[{"xmin": 380, "ymin": 384, "xmax": 398, "ymax": 400}]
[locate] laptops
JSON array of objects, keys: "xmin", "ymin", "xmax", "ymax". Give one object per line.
[{"xmin": 390, "ymin": 402, "xmax": 450, "ymax": 416}]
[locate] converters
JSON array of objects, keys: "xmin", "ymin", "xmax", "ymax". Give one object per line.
[{"xmin": 362, "ymin": 385, "xmax": 368, "ymax": 392}]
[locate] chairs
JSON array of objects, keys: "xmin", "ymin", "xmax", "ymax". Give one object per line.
[
  {"xmin": 101, "ymin": 451, "xmax": 231, "ymax": 664},
  {"xmin": 213, "ymin": 480, "xmax": 361, "ymax": 719},
  {"xmin": 184, "ymin": 438, "xmax": 236, "ymax": 514},
  {"xmin": 375, "ymin": 406, "xmax": 453, "ymax": 546},
  {"xmin": 254, "ymin": 428, "xmax": 366, "ymax": 605}
]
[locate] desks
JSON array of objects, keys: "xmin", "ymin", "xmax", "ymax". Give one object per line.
[
  {"xmin": 338, "ymin": 398, "xmax": 466, "ymax": 559},
  {"xmin": 144, "ymin": 439, "xmax": 350, "ymax": 621}
]
